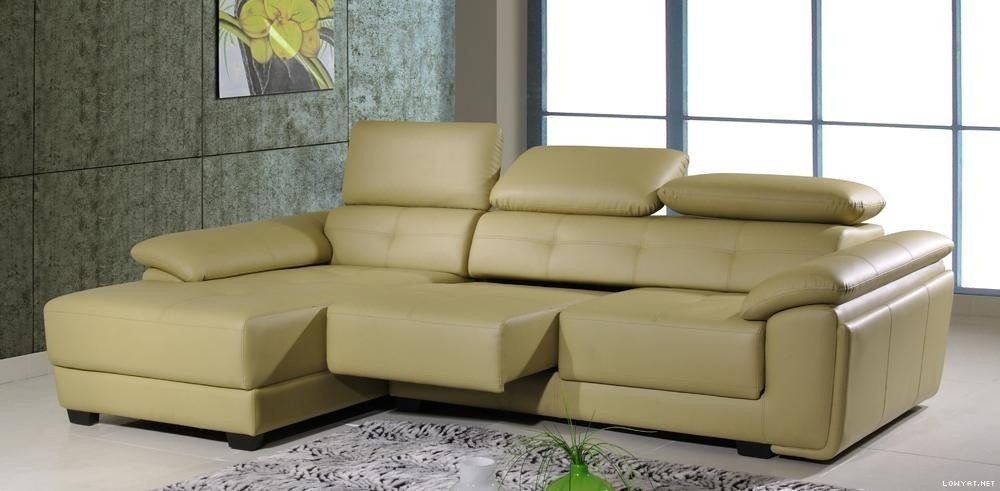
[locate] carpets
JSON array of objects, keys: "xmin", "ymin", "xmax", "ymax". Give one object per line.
[{"xmin": 151, "ymin": 415, "xmax": 865, "ymax": 491}]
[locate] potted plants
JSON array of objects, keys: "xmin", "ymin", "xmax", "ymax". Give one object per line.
[{"xmin": 487, "ymin": 397, "xmax": 656, "ymax": 491}]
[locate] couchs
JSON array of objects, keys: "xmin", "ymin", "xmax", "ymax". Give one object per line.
[{"xmin": 42, "ymin": 120, "xmax": 956, "ymax": 462}]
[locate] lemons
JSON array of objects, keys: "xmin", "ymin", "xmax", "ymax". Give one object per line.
[{"xmin": 239, "ymin": 0, "xmax": 320, "ymax": 64}]
